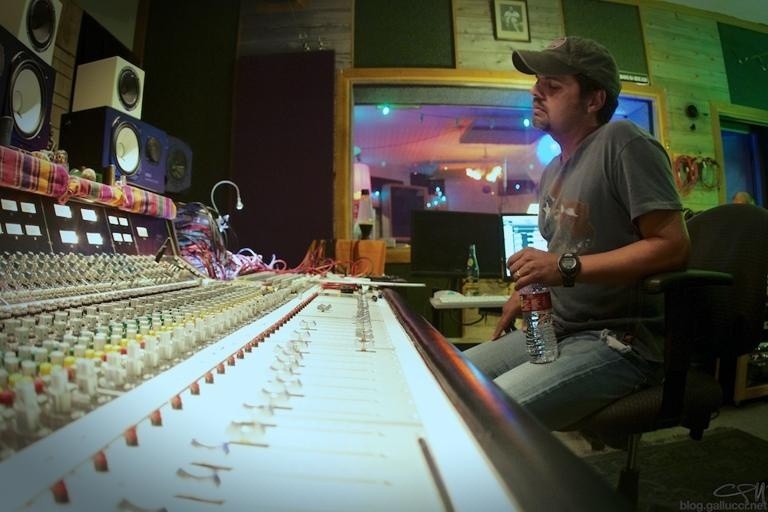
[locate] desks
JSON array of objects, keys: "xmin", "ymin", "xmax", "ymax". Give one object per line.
[{"xmin": 433, "ymin": 291, "xmax": 515, "ymax": 335}]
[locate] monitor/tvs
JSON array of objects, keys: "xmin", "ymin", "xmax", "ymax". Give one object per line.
[
  {"xmin": 498, "ymin": 213, "xmax": 549, "ymax": 282},
  {"xmin": 411, "ymin": 209, "xmax": 502, "ymax": 278}
]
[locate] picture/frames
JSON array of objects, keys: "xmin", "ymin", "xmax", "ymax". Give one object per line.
[{"xmin": 490, "ymin": 1, "xmax": 531, "ymax": 43}]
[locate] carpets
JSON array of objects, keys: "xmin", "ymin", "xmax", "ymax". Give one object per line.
[{"xmin": 582, "ymin": 426, "xmax": 766, "ymax": 511}]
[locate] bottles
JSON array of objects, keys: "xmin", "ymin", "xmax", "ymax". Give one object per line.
[
  {"xmin": 355, "ymin": 189, "xmax": 375, "ymax": 241},
  {"xmin": 464, "ymin": 242, "xmax": 480, "ymax": 296},
  {"xmin": 520, "ymin": 281, "xmax": 559, "ymax": 364}
]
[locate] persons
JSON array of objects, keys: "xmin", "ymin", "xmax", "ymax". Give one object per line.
[
  {"xmin": 734, "ymin": 191, "xmax": 768, "ymax": 212},
  {"xmin": 459, "ymin": 35, "xmax": 691, "ymax": 429},
  {"xmin": 354, "ymin": 186, "xmax": 380, "ymax": 239}
]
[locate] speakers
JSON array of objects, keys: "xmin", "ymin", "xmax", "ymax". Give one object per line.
[
  {"xmin": 58, "ymin": 106, "xmax": 168, "ymax": 194},
  {"xmin": 0, "ymin": 0, "xmax": 64, "ymax": 66},
  {"xmin": 381, "ymin": 184, "xmax": 428, "ymax": 242},
  {"xmin": 71, "ymin": 56, "xmax": 146, "ymax": 120},
  {"xmin": 0, "ymin": 26, "xmax": 58, "ymax": 154}
]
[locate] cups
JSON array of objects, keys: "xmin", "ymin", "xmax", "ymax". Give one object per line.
[
  {"xmin": 103, "ymin": 164, "xmax": 116, "ymax": 186},
  {"xmin": 358, "ymin": 223, "xmax": 373, "ymax": 239}
]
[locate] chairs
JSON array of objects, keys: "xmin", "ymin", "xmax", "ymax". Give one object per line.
[{"xmin": 569, "ymin": 198, "xmax": 767, "ymax": 510}]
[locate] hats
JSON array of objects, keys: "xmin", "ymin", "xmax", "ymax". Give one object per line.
[{"xmin": 512, "ymin": 36, "xmax": 622, "ymax": 100}]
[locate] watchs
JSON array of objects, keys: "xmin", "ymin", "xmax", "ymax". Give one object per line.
[{"xmin": 557, "ymin": 252, "xmax": 582, "ymax": 287}]
